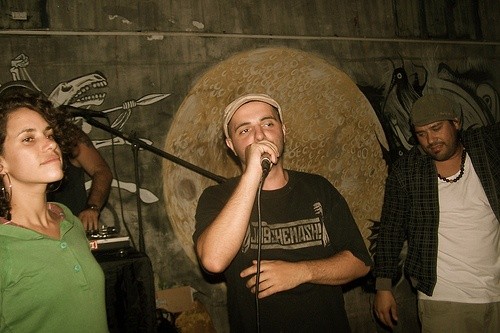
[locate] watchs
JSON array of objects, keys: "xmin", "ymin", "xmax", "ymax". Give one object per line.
[{"xmin": 84, "ymin": 204, "xmax": 100, "ymax": 213}]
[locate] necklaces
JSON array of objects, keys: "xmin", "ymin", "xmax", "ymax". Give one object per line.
[{"xmin": 437, "ymin": 149, "xmax": 466, "ymax": 182}]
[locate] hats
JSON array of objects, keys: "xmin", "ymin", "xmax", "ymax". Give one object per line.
[
  {"xmin": 410, "ymin": 94, "xmax": 461, "ymax": 127},
  {"xmin": 222, "ymin": 94, "xmax": 284, "ymax": 137}
]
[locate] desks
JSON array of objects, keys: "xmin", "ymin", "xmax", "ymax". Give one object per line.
[{"xmin": 91, "ymin": 246, "xmax": 155, "ymax": 333}]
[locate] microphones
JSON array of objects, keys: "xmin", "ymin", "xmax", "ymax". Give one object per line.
[
  {"xmin": 260, "ymin": 152, "xmax": 272, "ymax": 177},
  {"xmin": 58, "ymin": 104, "xmax": 108, "ymax": 118}
]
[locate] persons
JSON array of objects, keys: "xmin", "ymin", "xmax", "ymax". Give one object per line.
[
  {"xmin": 0, "ymin": 92, "xmax": 113, "ymax": 333},
  {"xmin": 192, "ymin": 93, "xmax": 373, "ymax": 333},
  {"xmin": 374, "ymin": 95, "xmax": 500, "ymax": 333}
]
